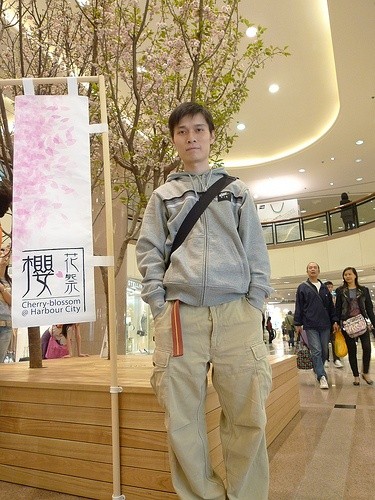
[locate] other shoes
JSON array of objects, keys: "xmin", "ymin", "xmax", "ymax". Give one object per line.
[
  {"xmin": 324, "ymin": 360, "xmax": 329, "ymax": 368},
  {"xmin": 353, "ymin": 378, "xmax": 360, "ymax": 386},
  {"xmin": 319, "ymin": 376, "xmax": 330, "ymax": 389},
  {"xmin": 361, "ymin": 374, "xmax": 373, "ymax": 385},
  {"xmin": 333, "ymin": 360, "xmax": 345, "ymax": 368}
]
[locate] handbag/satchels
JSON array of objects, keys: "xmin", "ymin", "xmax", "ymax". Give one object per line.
[
  {"xmin": 342, "ymin": 314, "xmax": 368, "ymax": 338},
  {"xmin": 45, "ymin": 337, "xmax": 69, "ymax": 359},
  {"xmin": 296, "ymin": 332, "xmax": 313, "ymax": 370},
  {"xmin": 291, "ymin": 326, "xmax": 296, "ymax": 332},
  {"xmin": 333, "ymin": 331, "xmax": 347, "ymax": 358}
]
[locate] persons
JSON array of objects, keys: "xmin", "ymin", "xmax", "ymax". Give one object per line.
[
  {"xmin": 0, "ymin": 252, "xmax": 12, "ymax": 363},
  {"xmin": 294, "ymin": 262, "xmax": 338, "ymax": 389},
  {"xmin": 339, "ymin": 192, "xmax": 353, "ymax": 232},
  {"xmin": 334, "ymin": 267, "xmax": 375, "ymax": 385},
  {"xmin": 283, "ymin": 311, "xmax": 302, "ymax": 347},
  {"xmin": 324, "ymin": 281, "xmax": 344, "ymax": 368},
  {"xmin": 266, "ymin": 316, "xmax": 273, "ymax": 343},
  {"xmin": 41, "ymin": 324, "xmax": 90, "ymax": 358},
  {"xmin": 135, "ymin": 102, "xmax": 276, "ymax": 500}
]
